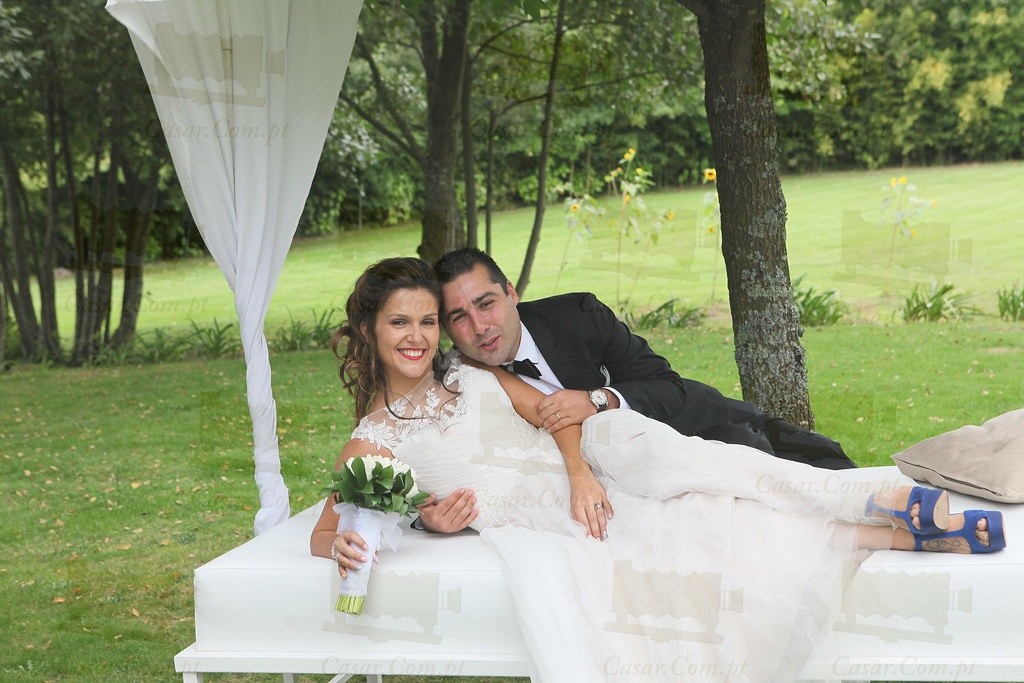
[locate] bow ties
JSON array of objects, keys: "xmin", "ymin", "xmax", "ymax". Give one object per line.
[{"xmin": 499, "ymin": 358, "xmax": 542, "ymax": 380}]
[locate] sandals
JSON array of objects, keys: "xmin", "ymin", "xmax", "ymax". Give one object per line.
[
  {"xmin": 913, "ymin": 510, "xmax": 1007, "ymax": 554},
  {"xmin": 865, "ymin": 486, "xmax": 949, "ymax": 535}
]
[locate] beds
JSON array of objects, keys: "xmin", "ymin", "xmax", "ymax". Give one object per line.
[{"xmin": 173, "ymin": 465, "xmax": 1024, "ymax": 683}]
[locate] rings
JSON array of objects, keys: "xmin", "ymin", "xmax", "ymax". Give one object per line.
[
  {"xmin": 336, "ymin": 553, "xmax": 340, "ymax": 560},
  {"xmin": 595, "ymin": 504, "xmax": 603, "ymax": 510},
  {"xmin": 554, "ymin": 412, "xmax": 561, "ymax": 420}
]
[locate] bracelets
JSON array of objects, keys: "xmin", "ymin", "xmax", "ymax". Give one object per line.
[{"xmin": 331, "ymin": 535, "xmax": 340, "ymax": 559}]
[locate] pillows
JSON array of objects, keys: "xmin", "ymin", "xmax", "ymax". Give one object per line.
[{"xmin": 889, "ymin": 407, "xmax": 1024, "ymax": 504}]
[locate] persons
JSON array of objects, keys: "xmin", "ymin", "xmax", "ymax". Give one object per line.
[
  {"xmin": 409, "ymin": 248, "xmax": 861, "ymax": 535},
  {"xmin": 309, "ymin": 253, "xmax": 1008, "ymax": 580}
]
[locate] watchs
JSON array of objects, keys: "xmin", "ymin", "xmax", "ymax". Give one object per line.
[{"xmin": 587, "ymin": 387, "xmax": 608, "ymax": 414}]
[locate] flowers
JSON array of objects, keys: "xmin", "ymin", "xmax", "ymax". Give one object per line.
[{"xmin": 316, "ymin": 454, "xmax": 431, "ymax": 613}]
